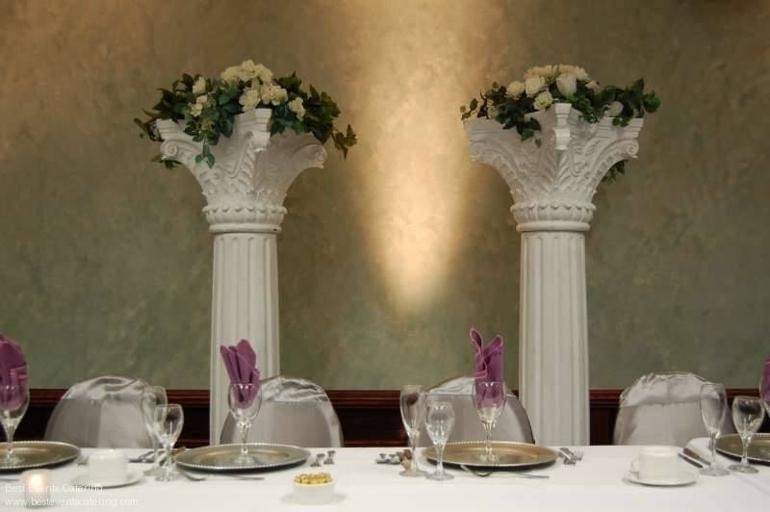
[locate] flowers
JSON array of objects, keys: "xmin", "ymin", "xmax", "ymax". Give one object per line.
[
  {"xmin": 133, "ymin": 60, "xmax": 358, "ymax": 172},
  {"xmin": 458, "ymin": 65, "xmax": 661, "ymax": 185}
]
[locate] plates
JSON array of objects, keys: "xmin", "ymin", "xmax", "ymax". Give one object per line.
[
  {"xmin": 174, "ymin": 444, "xmax": 312, "ymax": 472},
  {"xmin": 627, "ymin": 467, "xmax": 697, "ymax": 487},
  {"xmin": 420, "ymin": 440, "xmax": 557, "ymax": 470},
  {"xmin": 715, "ymin": 432, "xmax": 770, "ymax": 465},
  {"xmin": 72, "ymin": 472, "xmax": 144, "ymax": 489},
  {"xmin": 0, "ymin": 440, "xmax": 82, "ymax": 472}
]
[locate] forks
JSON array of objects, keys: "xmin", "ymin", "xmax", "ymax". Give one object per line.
[
  {"xmin": 389, "ymin": 453, "xmax": 400, "ymax": 466},
  {"xmin": 141, "ymin": 450, "xmax": 166, "ymax": 464},
  {"xmin": 375, "ymin": 452, "xmax": 387, "ymax": 465},
  {"xmin": 459, "ymin": 462, "xmax": 550, "ymax": 479},
  {"xmin": 129, "ymin": 449, "xmax": 154, "ymax": 463},
  {"xmin": 324, "ymin": 450, "xmax": 336, "ymax": 465},
  {"xmin": 555, "ymin": 450, "xmax": 576, "ymax": 466},
  {"xmin": 560, "ymin": 446, "xmax": 585, "ymax": 461}
]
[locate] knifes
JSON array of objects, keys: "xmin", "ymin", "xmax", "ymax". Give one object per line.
[
  {"xmin": 682, "ymin": 446, "xmax": 711, "ymax": 467},
  {"xmin": 310, "ymin": 453, "xmax": 325, "ymax": 469},
  {"xmin": 158, "ymin": 448, "xmax": 176, "ymax": 467},
  {"xmin": 674, "ymin": 452, "xmax": 703, "ymax": 470},
  {"xmin": 396, "ymin": 450, "xmax": 407, "ymax": 468},
  {"xmin": 404, "ymin": 449, "xmax": 414, "ymax": 464}
]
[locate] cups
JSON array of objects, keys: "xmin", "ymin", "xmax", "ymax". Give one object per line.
[
  {"xmin": 0, "ymin": 384, "xmax": 31, "ymax": 465},
  {"xmin": 140, "ymin": 386, "xmax": 168, "ymax": 477},
  {"xmin": 472, "ymin": 382, "xmax": 507, "ymax": 462},
  {"xmin": 400, "ymin": 384, "xmax": 428, "ymax": 477},
  {"xmin": 424, "ymin": 401, "xmax": 454, "ymax": 482},
  {"xmin": 759, "ymin": 361, "xmax": 770, "ymax": 421},
  {"xmin": 700, "ymin": 383, "xmax": 728, "ymax": 477},
  {"xmin": 629, "ymin": 450, "xmax": 678, "ymax": 481},
  {"xmin": 152, "ymin": 403, "xmax": 185, "ymax": 481},
  {"xmin": 228, "ymin": 384, "xmax": 263, "ymax": 464},
  {"xmin": 89, "ymin": 452, "xmax": 129, "ymax": 482},
  {"xmin": 730, "ymin": 395, "xmax": 765, "ymax": 475}
]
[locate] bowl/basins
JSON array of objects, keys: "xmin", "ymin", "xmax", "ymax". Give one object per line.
[{"xmin": 292, "ymin": 479, "xmax": 335, "ymax": 505}]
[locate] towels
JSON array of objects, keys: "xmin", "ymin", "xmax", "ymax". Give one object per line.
[
  {"xmin": 220, "ymin": 339, "xmax": 259, "ymax": 407},
  {"xmin": 469, "ymin": 326, "xmax": 504, "ymax": 407},
  {"xmin": 0, "ymin": 335, "xmax": 28, "ymax": 411}
]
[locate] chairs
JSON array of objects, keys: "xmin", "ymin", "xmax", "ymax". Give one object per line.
[
  {"xmin": 408, "ymin": 375, "xmax": 535, "ymax": 446},
  {"xmin": 219, "ymin": 375, "xmax": 343, "ymax": 448},
  {"xmin": 42, "ymin": 376, "xmax": 157, "ymax": 448},
  {"xmin": 614, "ymin": 371, "xmax": 737, "ymax": 445}
]
